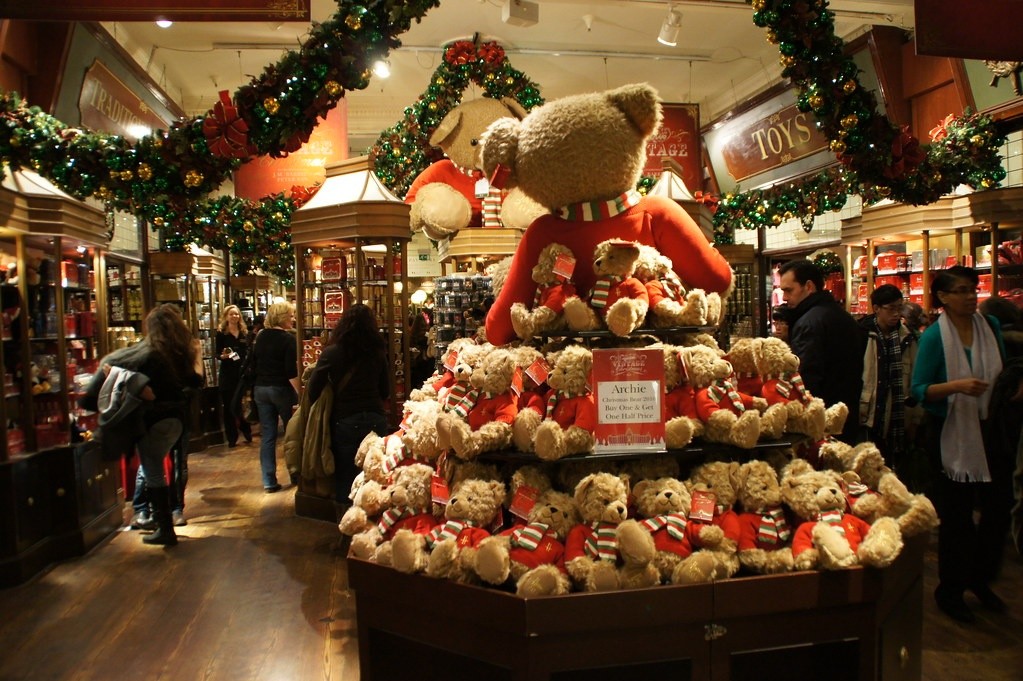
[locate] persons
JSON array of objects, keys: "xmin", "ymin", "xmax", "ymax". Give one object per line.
[
  {"xmin": 910, "ymin": 265, "xmax": 1011, "ymax": 624},
  {"xmin": 463, "ymin": 296, "xmax": 494, "ymax": 330},
  {"xmin": 251, "ymin": 298, "xmax": 298, "ymax": 492},
  {"xmin": 773, "ymin": 259, "xmax": 1023, "ymax": 499},
  {"xmin": 214, "ymin": 303, "xmax": 254, "ymax": 449},
  {"xmin": 408, "ymin": 311, "xmax": 437, "ymax": 390},
  {"xmin": 309, "ymin": 303, "xmax": 393, "ymax": 559},
  {"xmin": 82, "ymin": 303, "xmax": 203, "ymax": 547}
]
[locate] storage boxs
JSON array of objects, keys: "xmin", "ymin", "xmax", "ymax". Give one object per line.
[
  {"xmin": 301, "ymin": 250, "xmax": 404, "ymax": 418},
  {"xmin": 849, "ymin": 237, "xmax": 1021, "ymax": 315},
  {"xmin": 5, "ymin": 258, "xmax": 100, "ymax": 454},
  {"xmin": 106, "ymin": 279, "xmax": 206, "ymax": 350}
]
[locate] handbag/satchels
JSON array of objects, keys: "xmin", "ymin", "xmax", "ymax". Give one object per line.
[{"xmin": 231, "ymin": 329, "xmax": 264, "ymax": 392}]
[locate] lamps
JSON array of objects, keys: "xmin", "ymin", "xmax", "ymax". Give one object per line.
[{"xmin": 658, "ymin": 3, "xmax": 682, "ymax": 45}]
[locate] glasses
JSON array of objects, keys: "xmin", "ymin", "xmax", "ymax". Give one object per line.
[
  {"xmin": 878, "ymin": 304, "xmax": 905, "ymax": 314},
  {"xmin": 773, "ymin": 320, "xmax": 788, "ymax": 328},
  {"xmin": 942, "ymin": 285, "xmax": 981, "ymax": 296}
]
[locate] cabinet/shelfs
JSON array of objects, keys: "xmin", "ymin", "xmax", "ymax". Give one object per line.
[
  {"xmin": 0, "ymin": 239, "xmax": 129, "ymax": 587},
  {"xmin": 294, "ymin": 238, "xmax": 411, "ymax": 526}
]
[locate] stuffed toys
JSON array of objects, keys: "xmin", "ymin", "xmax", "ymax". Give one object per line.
[
  {"xmin": 399, "ymin": 336, "xmax": 852, "ymax": 458},
  {"xmin": 480, "ymin": 80, "xmax": 739, "ymax": 347},
  {"xmin": 339, "ymin": 430, "xmax": 941, "ymax": 600},
  {"xmin": 509, "ymin": 236, "xmax": 727, "ymax": 339},
  {"xmin": 403, "ymin": 96, "xmax": 552, "ymax": 240}
]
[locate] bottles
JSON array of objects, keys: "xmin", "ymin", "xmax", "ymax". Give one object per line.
[{"xmin": 29, "ymin": 258, "xmax": 144, "ymax": 396}]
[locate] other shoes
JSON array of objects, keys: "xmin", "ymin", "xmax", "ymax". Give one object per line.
[
  {"xmin": 934, "ymin": 583, "xmax": 982, "ymax": 625},
  {"xmin": 290, "ymin": 472, "xmax": 298, "ymax": 486},
  {"xmin": 965, "ymin": 576, "xmax": 1008, "ymax": 617},
  {"xmin": 266, "ymin": 484, "xmax": 282, "ymax": 493},
  {"xmin": 172, "ymin": 513, "xmax": 187, "ymax": 526},
  {"xmin": 143, "ymin": 527, "xmax": 177, "ymax": 546},
  {"xmin": 228, "ymin": 439, "xmax": 236, "ymax": 448},
  {"xmin": 138, "ymin": 513, "xmax": 158, "ymax": 530},
  {"xmin": 239, "ymin": 423, "xmax": 252, "ymax": 442},
  {"xmin": 130, "ymin": 513, "xmax": 140, "ymax": 529}
]
